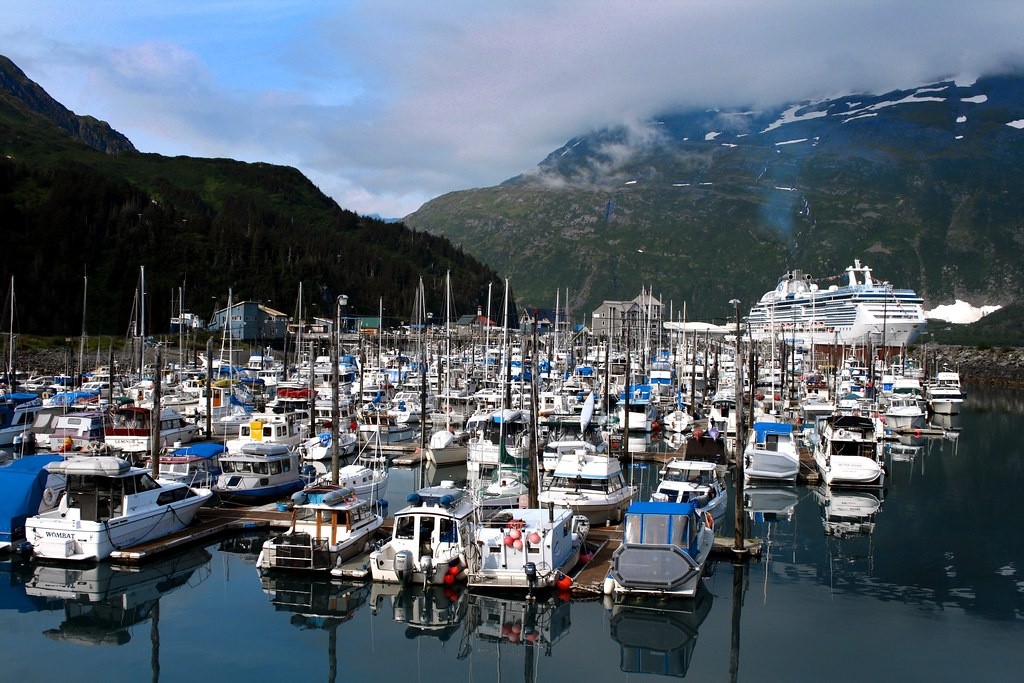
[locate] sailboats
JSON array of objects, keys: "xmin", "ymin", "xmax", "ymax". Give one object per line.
[{"xmin": 0, "ymin": 258, "xmax": 967, "ymax": 534}]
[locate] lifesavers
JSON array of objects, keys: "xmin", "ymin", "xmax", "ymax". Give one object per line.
[
  {"xmin": 42, "ymin": 392, "xmax": 48, "ymax": 398},
  {"xmin": 42, "ymin": 487, "xmax": 56, "ymax": 506},
  {"xmin": 837, "ymin": 428, "xmax": 846, "ymax": 437},
  {"xmin": 704, "ymin": 512, "xmax": 713, "ymax": 527},
  {"xmin": 727, "ymin": 379, "xmax": 731, "ymax": 383}
]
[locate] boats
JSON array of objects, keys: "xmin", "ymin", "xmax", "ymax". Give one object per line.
[
  {"xmin": 609, "ymin": 580, "xmax": 720, "ymax": 679},
  {"xmin": 604, "ymin": 501, "xmax": 715, "ymax": 598},
  {"xmin": 739, "ymin": 259, "xmax": 928, "ymax": 346},
  {"xmin": 256, "ymin": 566, "xmax": 374, "ymax": 632},
  {"xmin": 368, "ymin": 579, "xmax": 471, "ymax": 642},
  {"xmin": 255, "ymin": 483, "xmax": 392, "ymax": 578},
  {"xmin": 22, "ymin": 453, "xmax": 214, "ymax": 562},
  {"xmin": 369, "ymin": 486, "xmax": 591, "ymax": 592},
  {"xmin": 25, "ymin": 559, "xmax": 215, "ymax": 649},
  {"xmin": 455, "ymin": 577, "xmax": 571, "ymax": 663}
]
